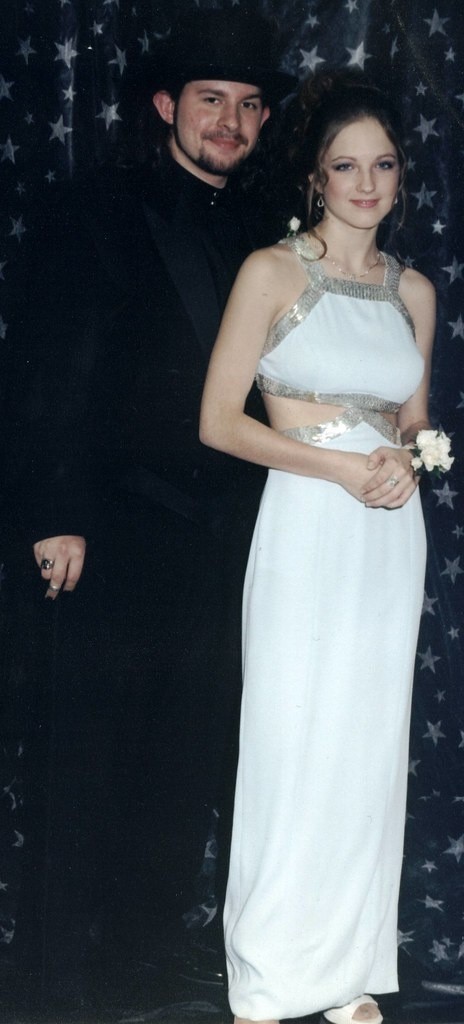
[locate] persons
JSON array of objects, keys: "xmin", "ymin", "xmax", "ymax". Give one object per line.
[
  {"xmin": 198, "ymin": 67, "xmax": 454, "ymax": 1024},
  {"xmin": 24, "ymin": 12, "xmax": 289, "ymax": 1012}
]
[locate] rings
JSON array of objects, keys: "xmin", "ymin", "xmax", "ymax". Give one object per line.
[
  {"xmin": 387, "ymin": 476, "xmax": 399, "ymax": 486},
  {"xmin": 40, "ymin": 558, "xmax": 53, "ymax": 569},
  {"xmin": 49, "ymin": 585, "xmax": 60, "ymax": 592}
]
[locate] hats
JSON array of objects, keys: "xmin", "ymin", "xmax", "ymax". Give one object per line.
[{"xmin": 156, "ymin": 28, "xmax": 296, "ymax": 107}]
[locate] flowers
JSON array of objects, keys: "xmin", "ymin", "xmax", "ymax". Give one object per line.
[{"xmin": 287, "ymin": 217, "xmax": 300, "ymax": 236}]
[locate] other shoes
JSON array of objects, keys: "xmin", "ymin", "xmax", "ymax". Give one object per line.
[
  {"xmin": 234, "ymin": 1015, "xmax": 280, "ymax": 1024},
  {"xmin": 323, "ymin": 996, "xmax": 384, "ymax": 1024}
]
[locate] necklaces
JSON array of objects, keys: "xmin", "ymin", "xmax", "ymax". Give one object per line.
[{"xmin": 312, "ymin": 240, "xmax": 382, "ymax": 281}]
